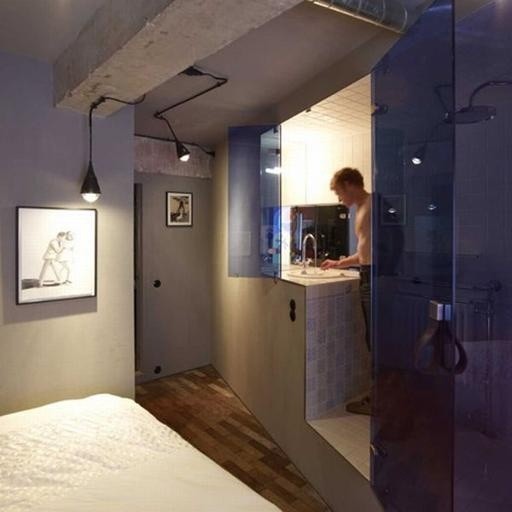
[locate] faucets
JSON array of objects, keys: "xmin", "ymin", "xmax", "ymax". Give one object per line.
[{"xmin": 300, "ymin": 233, "xmax": 317, "ymax": 273}]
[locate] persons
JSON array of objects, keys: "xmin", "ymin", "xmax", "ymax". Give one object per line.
[
  {"xmin": 318, "ymin": 168, "xmax": 404, "ymax": 415},
  {"xmin": 39, "ymin": 232, "xmax": 64, "ymax": 286}
]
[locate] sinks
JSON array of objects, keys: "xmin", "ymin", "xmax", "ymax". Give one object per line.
[{"xmin": 286, "ymin": 268, "xmax": 343, "ymax": 278}]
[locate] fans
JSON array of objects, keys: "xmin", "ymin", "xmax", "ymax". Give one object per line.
[{"xmin": 309, "ymin": 0, "xmax": 408, "ymax": 35}]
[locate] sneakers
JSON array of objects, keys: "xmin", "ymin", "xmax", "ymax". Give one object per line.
[{"xmin": 345, "ymin": 393, "xmax": 371, "ymax": 414}]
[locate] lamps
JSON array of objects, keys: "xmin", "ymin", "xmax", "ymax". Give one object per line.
[{"xmin": 82, "ymin": 67, "xmax": 228, "ymax": 203}]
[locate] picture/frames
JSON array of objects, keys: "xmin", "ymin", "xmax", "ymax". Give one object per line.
[
  {"xmin": 377, "ymin": 193, "xmax": 408, "ymax": 225},
  {"xmin": 16, "ymin": 191, "xmax": 193, "ymax": 306}
]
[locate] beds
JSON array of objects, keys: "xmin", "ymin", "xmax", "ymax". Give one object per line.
[{"xmin": 0, "ymin": 394, "xmax": 285, "ymax": 512}]
